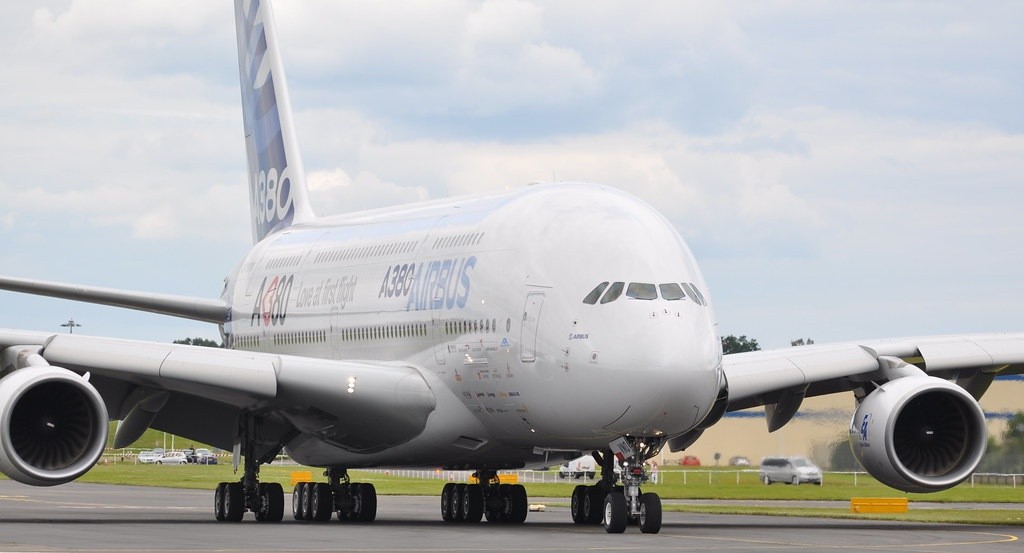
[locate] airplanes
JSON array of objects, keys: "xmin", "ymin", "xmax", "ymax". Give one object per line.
[{"xmin": 1, "ymin": 0, "xmax": 1024, "ymax": 533}]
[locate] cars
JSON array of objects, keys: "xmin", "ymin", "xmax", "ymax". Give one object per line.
[
  {"xmin": 137, "ymin": 451, "xmax": 156, "ymax": 463},
  {"xmin": 728, "ymin": 457, "xmax": 750, "ymax": 466},
  {"xmin": 184, "ymin": 451, "xmax": 196, "ymax": 462},
  {"xmin": 679, "ymin": 456, "xmax": 700, "ymax": 465}
]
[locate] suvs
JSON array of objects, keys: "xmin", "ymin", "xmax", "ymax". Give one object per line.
[
  {"xmin": 153, "ymin": 448, "xmax": 165, "ymax": 457},
  {"xmin": 196, "ymin": 449, "xmax": 217, "ymax": 464}
]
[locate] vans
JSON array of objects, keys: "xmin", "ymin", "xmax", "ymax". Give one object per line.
[
  {"xmin": 761, "ymin": 457, "xmax": 821, "ymax": 485},
  {"xmin": 558, "ymin": 455, "xmax": 596, "ymax": 478},
  {"xmin": 154, "ymin": 452, "xmax": 188, "ymax": 465}
]
